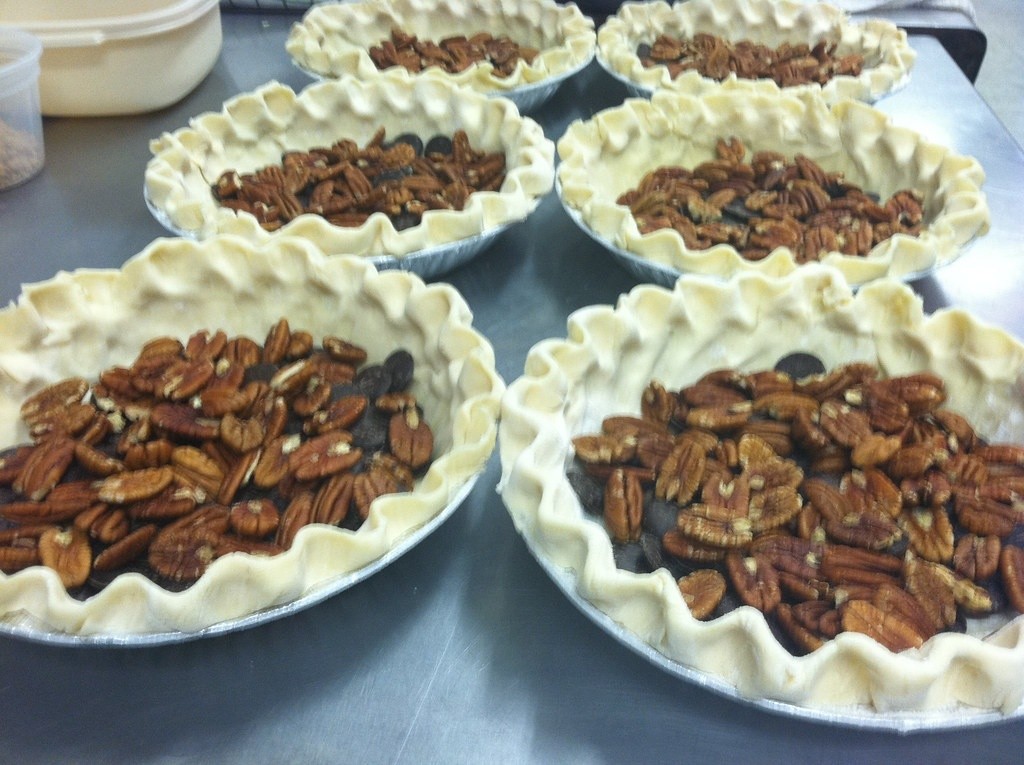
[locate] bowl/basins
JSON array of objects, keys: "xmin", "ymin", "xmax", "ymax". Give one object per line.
[{"xmin": 0, "ymin": 1, "xmax": 223, "ymax": 118}]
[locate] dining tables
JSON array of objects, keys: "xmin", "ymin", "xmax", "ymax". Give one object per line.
[{"xmin": 3, "ymin": 4, "xmax": 1024, "ymax": 761}]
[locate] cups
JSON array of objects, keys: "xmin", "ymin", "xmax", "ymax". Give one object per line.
[{"xmin": 0, "ymin": 28, "xmax": 48, "ymax": 194}]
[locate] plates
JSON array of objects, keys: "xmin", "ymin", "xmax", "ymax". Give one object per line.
[
  {"xmin": 556, "ymin": 101, "xmax": 993, "ymax": 302},
  {"xmin": 0, "ymin": 229, "xmax": 499, "ymax": 650},
  {"xmin": 289, "ymin": 0, "xmax": 598, "ymax": 117},
  {"xmin": 502, "ymin": 263, "xmax": 1024, "ymax": 735},
  {"xmin": 143, "ymin": 74, "xmax": 556, "ymax": 287},
  {"xmin": 597, "ymin": 1, "xmax": 917, "ymax": 108}
]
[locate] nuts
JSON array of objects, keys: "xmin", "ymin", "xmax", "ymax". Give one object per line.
[
  {"xmin": 366, "ymin": 20, "xmax": 549, "ymax": 77},
  {"xmin": 2, "ymin": 317, "xmax": 438, "ymax": 593},
  {"xmin": 571, "ymin": 353, "xmax": 1023, "ymax": 651},
  {"xmin": 634, "ymin": 29, "xmax": 869, "ymax": 92},
  {"xmin": 616, "ymin": 136, "xmax": 925, "ymax": 267},
  {"xmin": 211, "ymin": 124, "xmax": 507, "ymax": 233}
]
